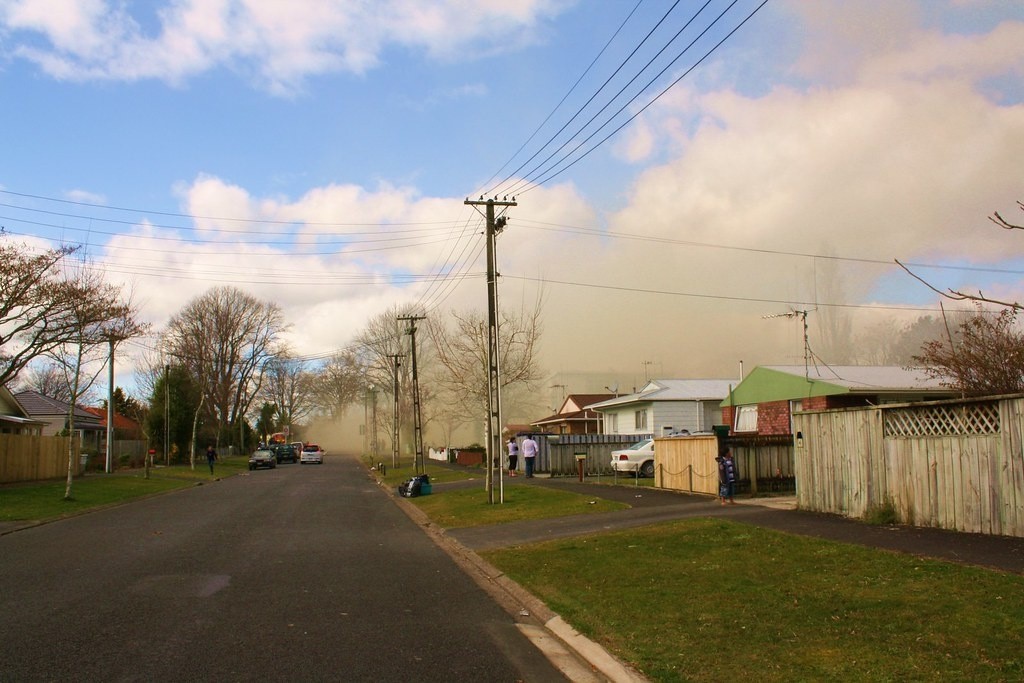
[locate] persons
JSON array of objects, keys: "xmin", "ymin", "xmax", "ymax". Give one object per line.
[
  {"xmin": 717, "ymin": 447, "xmax": 738, "ymax": 508},
  {"xmin": 522, "ymin": 434, "xmax": 539, "ymax": 479},
  {"xmin": 206, "ymin": 446, "xmax": 218, "ymax": 475},
  {"xmin": 507, "ymin": 437, "xmax": 519, "ymax": 477},
  {"xmin": 277, "ymin": 446, "xmax": 283, "ymax": 464}
]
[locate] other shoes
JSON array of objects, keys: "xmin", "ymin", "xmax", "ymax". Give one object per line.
[
  {"xmin": 730, "ymin": 500, "xmax": 737, "ymax": 505},
  {"xmin": 722, "ymin": 502, "xmax": 726, "ymax": 507},
  {"xmin": 526, "ymin": 475, "xmax": 534, "ymax": 478},
  {"xmin": 509, "ymin": 474, "xmax": 518, "ymax": 476}
]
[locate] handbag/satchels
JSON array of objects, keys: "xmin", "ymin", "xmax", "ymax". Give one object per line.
[{"xmin": 720, "ymin": 485, "xmax": 729, "ymax": 496}]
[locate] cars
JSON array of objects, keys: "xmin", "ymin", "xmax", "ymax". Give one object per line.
[
  {"xmin": 248, "ymin": 449, "xmax": 277, "ymax": 471},
  {"xmin": 299, "ymin": 443, "xmax": 324, "ymax": 464},
  {"xmin": 277, "ymin": 442, "xmax": 304, "ymax": 464},
  {"xmin": 611, "ymin": 438, "xmax": 656, "ymax": 478}
]
